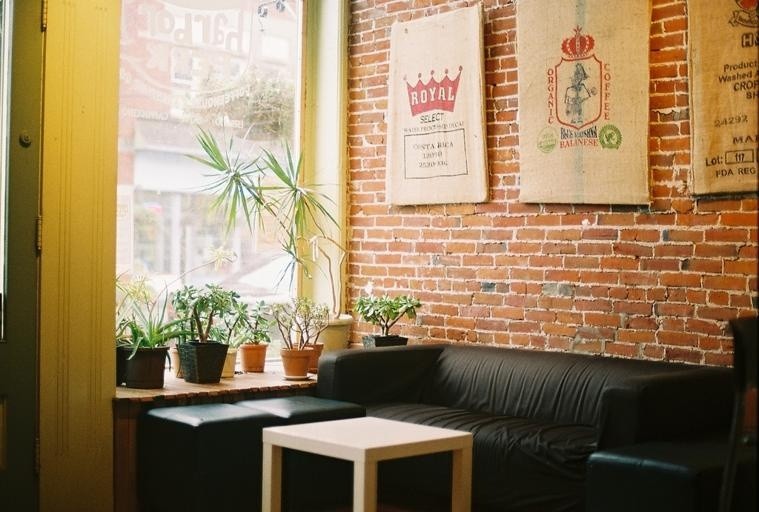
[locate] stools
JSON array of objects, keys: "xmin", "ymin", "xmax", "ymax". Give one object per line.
[
  {"xmin": 261, "ymin": 414, "xmax": 473, "ymax": 511},
  {"xmin": 138, "ymin": 394, "xmax": 365, "ymax": 511}
]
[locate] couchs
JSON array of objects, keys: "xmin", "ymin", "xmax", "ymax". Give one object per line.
[{"xmin": 316, "ymin": 341, "xmax": 745, "ymax": 510}]
